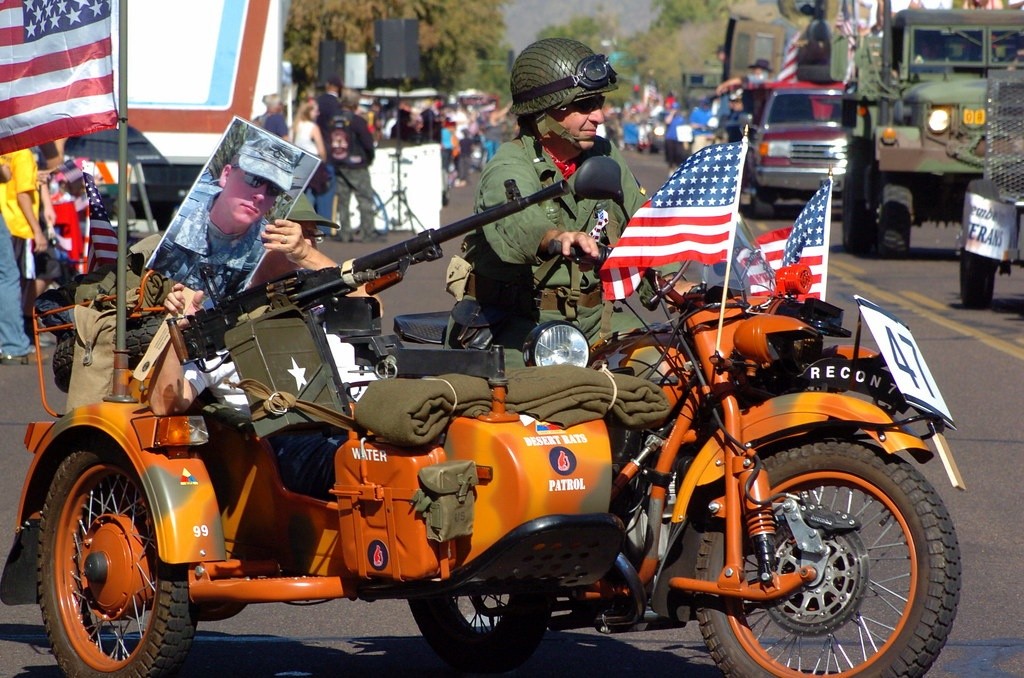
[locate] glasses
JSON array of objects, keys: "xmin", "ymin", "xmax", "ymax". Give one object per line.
[
  {"xmin": 571, "ymin": 54, "xmax": 618, "ymax": 91},
  {"xmin": 301, "ymin": 229, "xmax": 326, "ymax": 244},
  {"xmin": 231, "ymin": 162, "xmax": 284, "ymax": 197}
]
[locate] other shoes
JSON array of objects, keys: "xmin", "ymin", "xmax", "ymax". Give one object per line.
[
  {"xmin": 362, "ymin": 230, "xmax": 388, "ymax": 243},
  {"xmin": 0, "ymin": 349, "xmax": 50, "ymax": 366}
]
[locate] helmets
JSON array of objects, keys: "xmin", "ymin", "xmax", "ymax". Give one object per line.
[{"xmin": 507, "ymin": 38, "xmax": 620, "ymax": 116}]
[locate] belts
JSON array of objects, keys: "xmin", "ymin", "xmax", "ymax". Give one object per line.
[{"xmin": 464, "ymin": 274, "xmax": 604, "ymax": 311}]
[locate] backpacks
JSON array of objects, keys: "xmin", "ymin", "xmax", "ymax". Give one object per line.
[{"xmin": 326, "ymin": 111, "xmax": 357, "ymax": 163}]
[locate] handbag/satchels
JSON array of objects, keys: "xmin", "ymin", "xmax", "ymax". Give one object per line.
[{"xmin": 445, "ymin": 255, "xmax": 474, "ymax": 303}]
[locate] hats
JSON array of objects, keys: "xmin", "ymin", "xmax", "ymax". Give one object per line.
[
  {"xmin": 288, "ymin": 193, "xmax": 340, "ymax": 230},
  {"xmin": 231, "ymin": 126, "xmax": 302, "ymax": 192},
  {"xmin": 747, "ymin": 58, "xmax": 773, "ymax": 73}
]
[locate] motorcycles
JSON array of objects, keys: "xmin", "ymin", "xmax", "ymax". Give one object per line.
[{"xmin": 0, "ymin": 1, "xmax": 968, "ymax": 676}]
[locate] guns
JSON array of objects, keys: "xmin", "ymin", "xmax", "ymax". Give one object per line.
[{"xmin": 165, "ymin": 179, "xmax": 571, "ymax": 438}]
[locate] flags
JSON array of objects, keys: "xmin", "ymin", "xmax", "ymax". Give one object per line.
[
  {"xmin": 599, "ymin": 141, "xmax": 743, "ymax": 301},
  {"xmin": 737, "ymin": 179, "xmax": 831, "ymax": 303},
  {"xmin": 83, "ymin": 171, "xmax": 119, "ymax": 274},
  {"xmin": 0, "ymin": 0, "xmax": 118, "ymax": 158}
]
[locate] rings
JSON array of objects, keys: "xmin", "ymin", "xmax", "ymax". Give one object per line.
[{"xmin": 284, "ymin": 237, "xmax": 288, "ymax": 243}]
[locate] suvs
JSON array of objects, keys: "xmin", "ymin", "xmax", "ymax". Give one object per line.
[{"xmin": 663, "ymin": 1, "xmax": 1024, "ymax": 311}]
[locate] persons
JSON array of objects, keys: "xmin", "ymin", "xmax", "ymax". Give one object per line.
[
  {"xmin": 716, "ymin": 59, "xmax": 773, "ymax": 96},
  {"xmin": 690, "ymin": 97, "xmax": 717, "ymax": 155},
  {"xmin": 472, "ymin": 37, "xmax": 699, "ymax": 369},
  {"xmin": 603, "ymin": 98, "xmax": 665, "ymax": 155},
  {"xmin": 255, "ymin": 75, "xmax": 387, "ymax": 244},
  {"xmin": 147, "ymin": 129, "xmax": 301, "ymax": 303},
  {"xmin": 917, "ymin": 39, "xmax": 953, "ymax": 73},
  {"xmin": 149, "ymin": 191, "xmax": 384, "ymax": 502},
  {"xmin": 385, "ymin": 88, "xmax": 520, "ymax": 211},
  {"xmin": 854, "ymin": 0, "xmax": 1024, "ymax": 36},
  {"xmin": 1008, "ymin": 48, "xmax": 1024, "ymax": 70},
  {"xmin": 664, "ymin": 102, "xmax": 687, "ymax": 178},
  {"xmin": 0, "ymin": 138, "xmax": 83, "ymax": 364}
]
